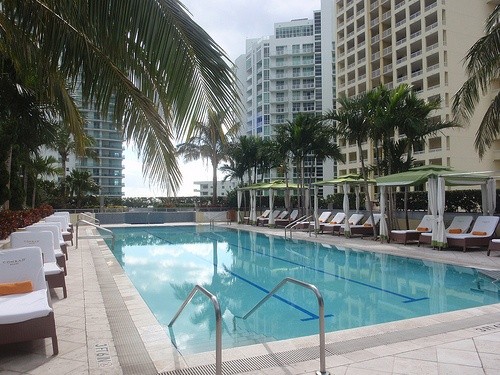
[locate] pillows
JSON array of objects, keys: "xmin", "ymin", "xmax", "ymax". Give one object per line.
[
  {"xmin": 417, "ymin": 228, "xmax": 428, "ymax": 231},
  {"xmin": 0, "ymin": 281, "xmax": 33, "ymax": 295},
  {"xmin": 449, "ymin": 229, "xmax": 461, "ymax": 234},
  {"xmin": 473, "ymin": 232, "xmax": 486, "ymax": 235}
]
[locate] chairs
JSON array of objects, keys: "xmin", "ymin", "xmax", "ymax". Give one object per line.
[
  {"xmin": 9, "ymin": 212, "xmax": 73, "ymax": 298},
  {"xmin": 297, "ymin": 212, "xmax": 381, "ymax": 239},
  {"xmin": 0, "ymin": 246, "xmax": 58, "ymax": 356},
  {"xmin": 391, "ymin": 215, "xmax": 500, "ymax": 256},
  {"xmin": 244, "ymin": 209, "xmax": 299, "ymax": 227}
]
